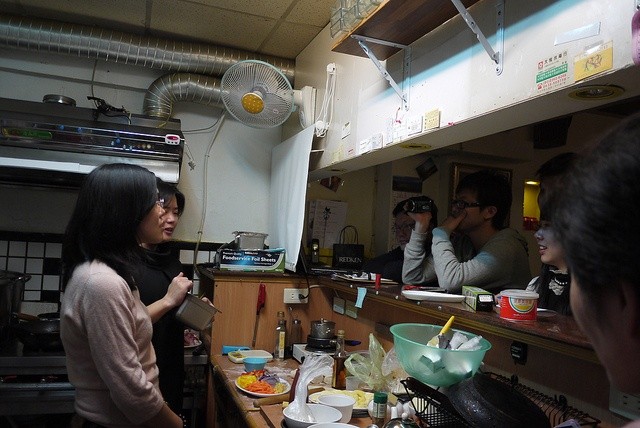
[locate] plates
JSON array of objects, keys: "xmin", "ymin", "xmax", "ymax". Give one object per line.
[
  {"xmin": 235, "ymin": 375, "xmax": 291, "ymax": 396},
  {"xmin": 402, "ymin": 290, "xmax": 465, "ymax": 302},
  {"xmin": 308, "ymin": 390, "xmax": 374, "ymax": 414}
]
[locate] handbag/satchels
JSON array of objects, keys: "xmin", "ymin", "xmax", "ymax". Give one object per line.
[{"xmin": 332, "ymin": 225, "xmax": 365, "ymax": 270}]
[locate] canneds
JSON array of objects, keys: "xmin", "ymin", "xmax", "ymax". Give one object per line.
[{"xmin": 371, "ymin": 392, "xmax": 387, "ymax": 428}]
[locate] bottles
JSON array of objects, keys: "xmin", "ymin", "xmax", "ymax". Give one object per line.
[
  {"xmin": 272, "ymin": 311, "xmax": 303, "ymax": 361},
  {"xmin": 372, "ymin": 393, "xmax": 387, "ymax": 428},
  {"xmin": 331, "ymin": 329, "xmax": 350, "ymax": 390}
]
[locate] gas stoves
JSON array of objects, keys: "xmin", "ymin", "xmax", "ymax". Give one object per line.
[{"xmin": 291, "ymin": 343, "xmax": 350, "ymax": 364}]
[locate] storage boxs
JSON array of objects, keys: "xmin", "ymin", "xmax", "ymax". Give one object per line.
[
  {"xmin": 215, "ymin": 247, "xmax": 287, "ymax": 273},
  {"xmin": 239, "ymin": 233, "xmax": 265, "ymax": 249}
]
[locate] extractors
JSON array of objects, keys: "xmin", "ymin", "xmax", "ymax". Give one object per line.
[{"xmin": 0, "ymin": 97, "xmax": 186, "ymax": 199}]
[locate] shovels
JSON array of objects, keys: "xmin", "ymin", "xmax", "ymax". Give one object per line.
[{"xmin": 427, "ymin": 316, "xmax": 454, "ymax": 348}]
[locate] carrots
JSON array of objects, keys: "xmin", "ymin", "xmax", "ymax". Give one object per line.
[{"xmin": 245, "ymin": 381, "xmax": 275, "ymax": 394}]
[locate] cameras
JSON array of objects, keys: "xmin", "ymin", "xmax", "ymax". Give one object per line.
[{"xmin": 406, "ymin": 200, "xmax": 434, "ymax": 213}]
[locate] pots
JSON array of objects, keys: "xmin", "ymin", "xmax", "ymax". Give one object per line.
[
  {"xmin": 0, "ymin": 269, "xmax": 61, "ymax": 347},
  {"xmin": 305, "ymin": 318, "xmax": 362, "ymax": 352},
  {"xmin": 399, "ymin": 372, "xmax": 552, "ymax": 428}
]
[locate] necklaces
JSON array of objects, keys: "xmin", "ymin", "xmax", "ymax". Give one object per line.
[{"xmin": 555, "ymin": 278, "xmax": 568, "ymax": 285}]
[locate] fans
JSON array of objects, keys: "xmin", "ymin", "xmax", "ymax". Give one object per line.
[
  {"xmin": 462, "ymin": 284, "xmax": 494, "ymax": 314},
  {"xmin": 217, "ymin": 58, "xmax": 319, "ymax": 131}
]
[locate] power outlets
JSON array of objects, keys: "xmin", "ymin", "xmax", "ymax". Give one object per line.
[
  {"xmin": 283, "ymin": 286, "xmax": 309, "ymax": 304},
  {"xmin": 333, "ymin": 297, "xmax": 345, "ymax": 314},
  {"xmin": 344, "ymin": 301, "xmax": 357, "ymax": 319},
  {"xmin": 328, "ymin": 62, "xmax": 335, "ymax": 74}
]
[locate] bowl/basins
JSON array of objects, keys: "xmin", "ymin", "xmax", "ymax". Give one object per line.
[
  {"xmin": 389, "ymin": 322, "xmax": 493, "ymax": 388},
  {"xmin": 43, "ymin": 94, "xmax": 76, "ymax": 106},
  {"xmin": 243, "ymin": 357, "xmax": 267, "ymax": 373},
  {"xmin": 499, "ymin": 290, "xmax": 539, "ymax": 321},
  {"xmin": 307, "ymin": 422, "xmax": 361, "ymax": 428},
  {"xmin": 317, "ymin": 393, "xmax": 356, "ymax": 423},
  {"xmin": 381, "ymin": 418, "xmax": 420, "ymax": 428},
  {"xmin": 232, "ymin": 231, "xmax": 268, "ymax": 250},
  {"xmin": 283, "ymin": 403, "xmax": 342, "ymax": 428}
]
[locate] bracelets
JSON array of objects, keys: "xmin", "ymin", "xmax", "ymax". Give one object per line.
[{"xmin": 179, "ymin": 414, "xmax": 187, "ymax": 428}]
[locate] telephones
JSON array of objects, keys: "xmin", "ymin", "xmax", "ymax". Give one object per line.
[{"xmin": 310, "ymin": 239, "xmax": 319, "ymax": 264}]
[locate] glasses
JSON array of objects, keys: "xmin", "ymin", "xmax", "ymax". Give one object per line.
[
  {"xmin": 451, "ymin": 200, "xmax": 481, "ymax": 208},
  {"xmin": 154, "ymin": 199, "xmax": 164, "ymax": 207},
  {"xmin": 392, "ymin": 219, "xmax": 415, "ymax": 233}
]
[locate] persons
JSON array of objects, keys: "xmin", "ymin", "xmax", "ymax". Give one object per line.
[
  {"xmin": 401, "ymin": 171, "xmax": 532, "ymax": 293},
  {"xmin": 537, "ymin": 152, "xmax": 580, "ymax": 211},
  {"xmin": 526, "ymin": 201, "xmax": 573, "ymax": 315},
  {"xmin": 364, "ymin": 196, "xmax": 438, "ymax": 281},
  {"xmin": 60, "ymin": 163, "xmax": 187, "ymax": 428},
  {"xmin": 548, "ymin": 114, "xmax": 640, "ymax": 428},
  {"xmin": 132, "ymin": 181, "xmax": 215, "ymax": 414}
]
